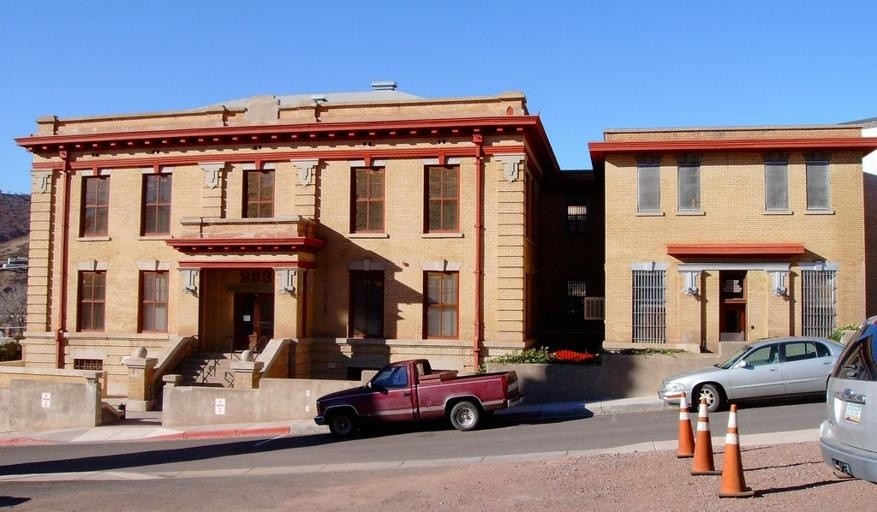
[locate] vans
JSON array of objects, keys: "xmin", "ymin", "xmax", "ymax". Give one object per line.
[{"xmin": 820, "ymin": 315, "xmax": 877, "ymax": 485}]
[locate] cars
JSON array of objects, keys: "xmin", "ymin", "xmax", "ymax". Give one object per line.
[{"xmin": 658, "ymin": 336, "xmax": 847, "ymax": 411}]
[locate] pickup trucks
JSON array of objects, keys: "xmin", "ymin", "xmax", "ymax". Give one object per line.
[{"xmin": 314, "ymin": 359, "xmax": 524, "ymax": 438}]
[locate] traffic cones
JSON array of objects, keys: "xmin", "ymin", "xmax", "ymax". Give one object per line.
[
  {"xmin": 715, "ymin": 403, "xmax": 758, "ymax": 498},
  {"xmin": 690, "ymin": 399, "xmax": 721, "ymax": 475},
  {"xmin": 676, "ymin": 391, "xmax": 697, "ymax": 458}
]
